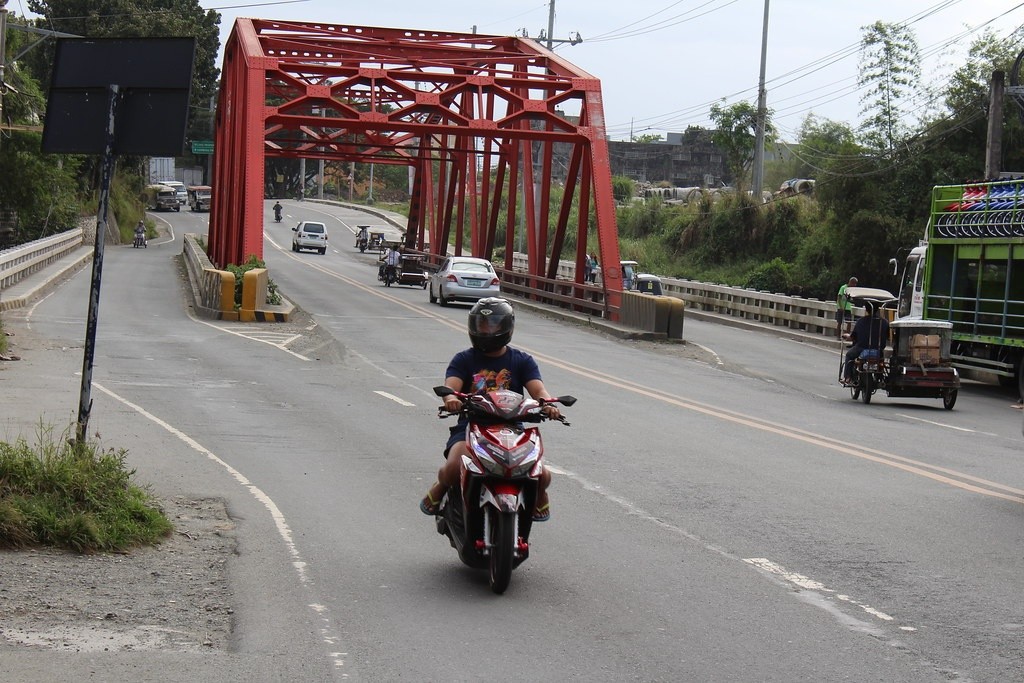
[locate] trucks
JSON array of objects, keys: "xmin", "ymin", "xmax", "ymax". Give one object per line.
[
  {"xmin": 147, "ymin": 184, "xmax": 180, "ymax": 213},
  {"xmin": 896, "ymin": 179, "xmax": 1023, "ymax": 405}
]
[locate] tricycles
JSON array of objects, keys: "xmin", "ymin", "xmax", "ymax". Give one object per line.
[
  {"xmin": 837, "ymin": 286, "xmax": 959, "ymax": 411},
  {"xmin": 354, "ymin": 226, "xmax": 387, "ymax": 252},
  {"xmin": 133, "ymin": 229, "xmax": 148, "ymax": 248},
  {"xmin": 378, "ymin": 240, "xmax": 428, "ymax": 290}
]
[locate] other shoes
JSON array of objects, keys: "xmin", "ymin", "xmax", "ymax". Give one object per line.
[{"xmin": 836, "ymin": 337, "xmax": 843, "ymax": 341}]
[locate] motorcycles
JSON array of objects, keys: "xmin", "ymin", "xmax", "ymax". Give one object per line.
[
  {"xmin": 273, "ymin": 206, "xmax": 283, "ymax": 223},
  {"xmin": 432, "ymin": 385, "xmax": 580, "ymax": 594}
]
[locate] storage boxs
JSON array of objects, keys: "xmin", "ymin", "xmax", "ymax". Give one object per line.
[{"xmin": 911, "ymin": 333, "xmax": 940, "ymax": 364}]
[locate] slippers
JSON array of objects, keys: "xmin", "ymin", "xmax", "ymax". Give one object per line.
[
  {"xmin": 531, "ymin": 493, "xmax": 550, "ymax": 522},
  {"xmin": 419, "ymin": 481, "xmax": 443, "ymax": 516}
]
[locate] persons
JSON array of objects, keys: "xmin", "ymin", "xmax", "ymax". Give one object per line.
[
  {"xmin": 838, "ymin": 300, "xmax": 890, "ymax": 384},
  {"xmin": 134, "ymin": 221, "xmax": 147, "ymax": 243},
  {"xmin": 583, "ymin": 254, "xmax": 591, "ymax": 282},
  {"xmin": 415, "ymin": 295, "xmax": 563, "ymax": 523},
  {"xmin": 620, "ymin": 265, "xmax": 630, "ymax": 279},
  {"xmin": 353, "ymin": 227, "xmax": 368, "ymax": 248},
  {"xmin": 273, "ymin": 201, "xmax": 282, "ymax": 221},
  {"xmin": 834, "ymin": 277, "xmax": 859, "ymax": 339},
  {"xmin": 378, "ymin": 243, "xmax": 402, "ymax": 283},
  {"xmin": 589, "ymin": 252, "xmax": 599, "ymax": 283}
]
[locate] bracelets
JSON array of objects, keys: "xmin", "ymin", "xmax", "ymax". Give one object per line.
[{"xmin": 546, "ymin": 403, "xmax": 558, "ymax": 407}]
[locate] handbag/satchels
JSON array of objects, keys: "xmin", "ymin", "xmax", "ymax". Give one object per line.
[{"xmin": 590, "ymin": 268, "xmax": 597, "ymax": 274}]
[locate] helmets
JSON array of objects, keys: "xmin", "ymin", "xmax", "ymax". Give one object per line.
[
  {"xmin": 469, "ymin": 296, "xmax": 516, "ymax": 353},
  {"xmin": 139, "ymin": 220, "xmax": 143, "ymax": 227}
]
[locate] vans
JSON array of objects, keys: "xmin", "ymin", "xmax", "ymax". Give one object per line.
[
  {"xmin": 158, "ymin": 180, "xmax": 188, "ymax": 205},
  {"xmin": 186, "ymin": 185, "xmax": 211, "ymax": 211}
]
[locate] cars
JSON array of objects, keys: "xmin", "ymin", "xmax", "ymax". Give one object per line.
[
  {"xmin": 292, "ymin": 221, "xmax": 328, "ymax": 254},
  {"xmin": 636, "ymin": 273, "xmax": 664, "ymax": 296},
  {"xmin": 430, "ymin": 255, "xmax": 501, "ymax": 306}
]
